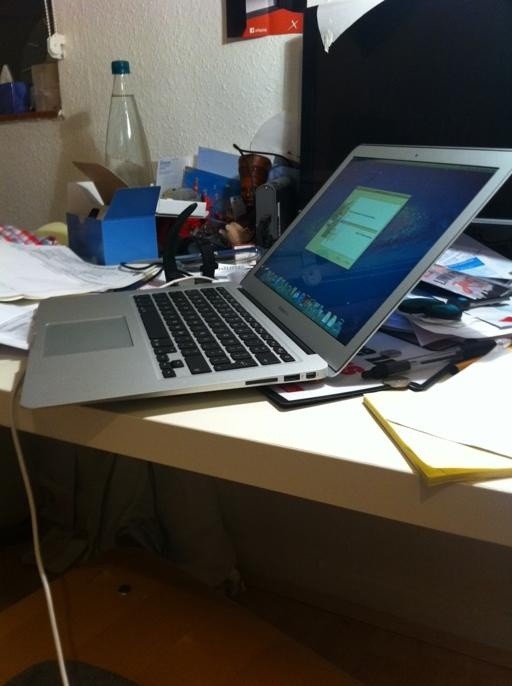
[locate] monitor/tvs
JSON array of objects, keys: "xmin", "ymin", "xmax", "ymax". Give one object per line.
[{"xmin": 300, "ymin": 0, "xmax": 512, "ymax": 248}]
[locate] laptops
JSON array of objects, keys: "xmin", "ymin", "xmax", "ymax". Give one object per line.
[{"xmin": 18, "ymin": 143, "xmax": 512, "ymax": 412}]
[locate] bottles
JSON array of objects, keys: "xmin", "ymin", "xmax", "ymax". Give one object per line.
[{"xmin": 107, "ymin": 65, "xmax": 159, "ymax": 196}]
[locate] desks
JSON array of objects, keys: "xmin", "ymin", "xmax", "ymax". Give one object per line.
[{"xmin": 0, "ymin": 344, "xmax": 512, "ymax": 549}]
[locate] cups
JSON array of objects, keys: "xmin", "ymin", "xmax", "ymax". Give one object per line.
[{"xmin": 10, "ymin": 79, "xmax": 26, "ymax": 112}]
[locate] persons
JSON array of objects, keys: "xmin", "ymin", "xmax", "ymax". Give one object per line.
[{"xmin": 238, "ymin": 153, "xmax": 273, "ymax": 235}]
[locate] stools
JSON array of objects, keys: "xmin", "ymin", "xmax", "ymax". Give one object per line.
[{"xmin": 0, "ymin": 545, "xmax": 366, "ymax": 686}]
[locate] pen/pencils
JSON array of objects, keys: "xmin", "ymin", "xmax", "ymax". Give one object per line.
[
  {"xmin": 124, "ymin": 247, "xmax": 255, "ymax": 263},
  {"xmin": 361, "ymin": 338, "xmax": 511, "ymax": 378}
]
